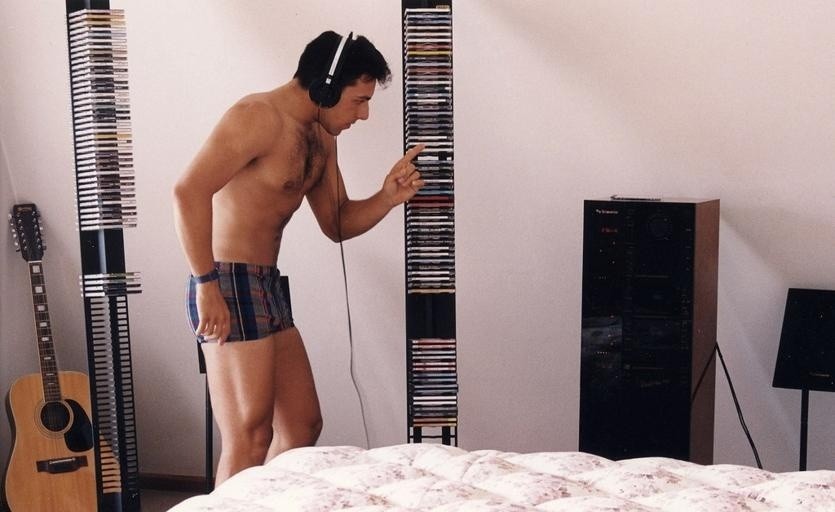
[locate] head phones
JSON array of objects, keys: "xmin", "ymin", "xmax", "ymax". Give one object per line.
[{"xmin": 308, "ymin": 31, "xmax": 353, "ymax": 108}]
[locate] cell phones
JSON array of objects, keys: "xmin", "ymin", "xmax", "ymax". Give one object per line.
[{"xmin": 611, "ymin": 195, "xmax": 661, "ymax": 201}]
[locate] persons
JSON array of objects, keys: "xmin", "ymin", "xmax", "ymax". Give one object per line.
[{"xmin": 169, "ymin": 29, "xmax": 427, "ymax": 487}]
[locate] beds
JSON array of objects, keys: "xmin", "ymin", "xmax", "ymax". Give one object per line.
[{"xmin": 164, "ymin": 441, "xmax": 833, "ymax": 512}]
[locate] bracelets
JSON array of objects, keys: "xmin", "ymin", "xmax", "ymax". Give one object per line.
[{"xmin": 194, "ymin": 268, "xmax": 219, "ymax": 288}]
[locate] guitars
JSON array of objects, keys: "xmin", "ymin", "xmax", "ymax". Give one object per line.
[{"xmin": 3, "ymin": 203, "xmax": 121, "ymax": 510}]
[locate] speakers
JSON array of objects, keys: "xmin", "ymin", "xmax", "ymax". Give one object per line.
[
  {"xmin": 772, "ymin": 288, "xmax": 835, "ymax": 392},
  {"xmin": 579, "ymin": 196, "xmax": 720, "ymax": 464}
]
